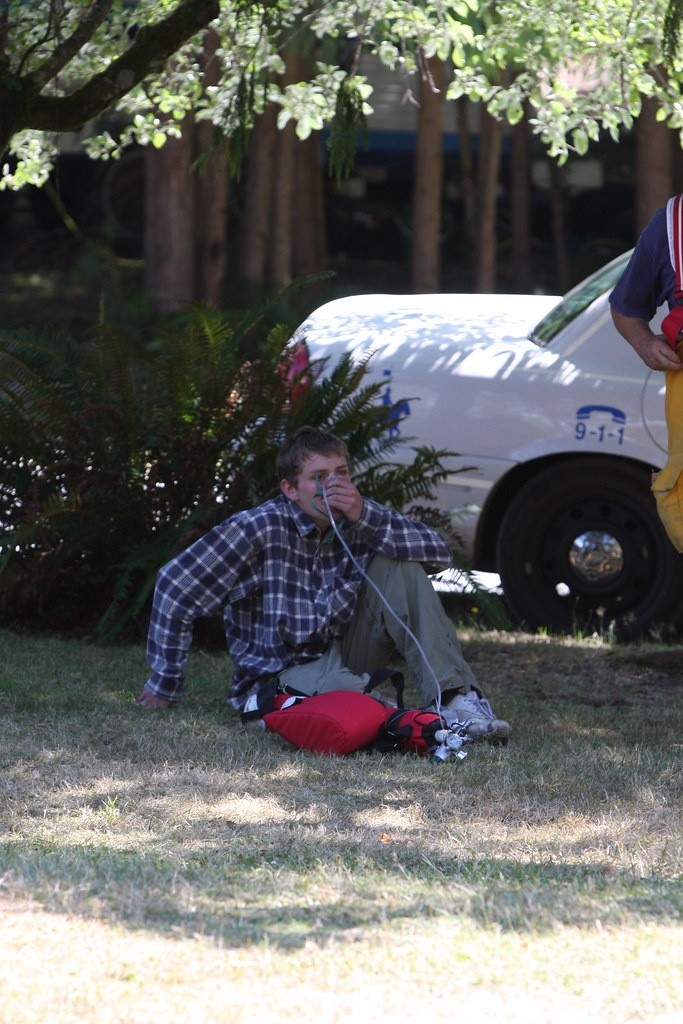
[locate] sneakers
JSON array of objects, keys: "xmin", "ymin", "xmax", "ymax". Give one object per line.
[{"xmin": 426, "ymin": 690, "xmax": 512, "ymax": 742}]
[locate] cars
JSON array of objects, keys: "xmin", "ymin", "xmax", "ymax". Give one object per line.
[{"xmin": 266, "ymin": 242, "xmax": 683, "ymax": 645}]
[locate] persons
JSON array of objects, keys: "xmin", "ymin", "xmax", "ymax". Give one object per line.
[
  {"xmin": 609, "ymin": 193, "xmax": 683, "ymax": 556},
  {"xmin": 138, "ymin": 426, "xmax": 511, "ymax": 745}
]
